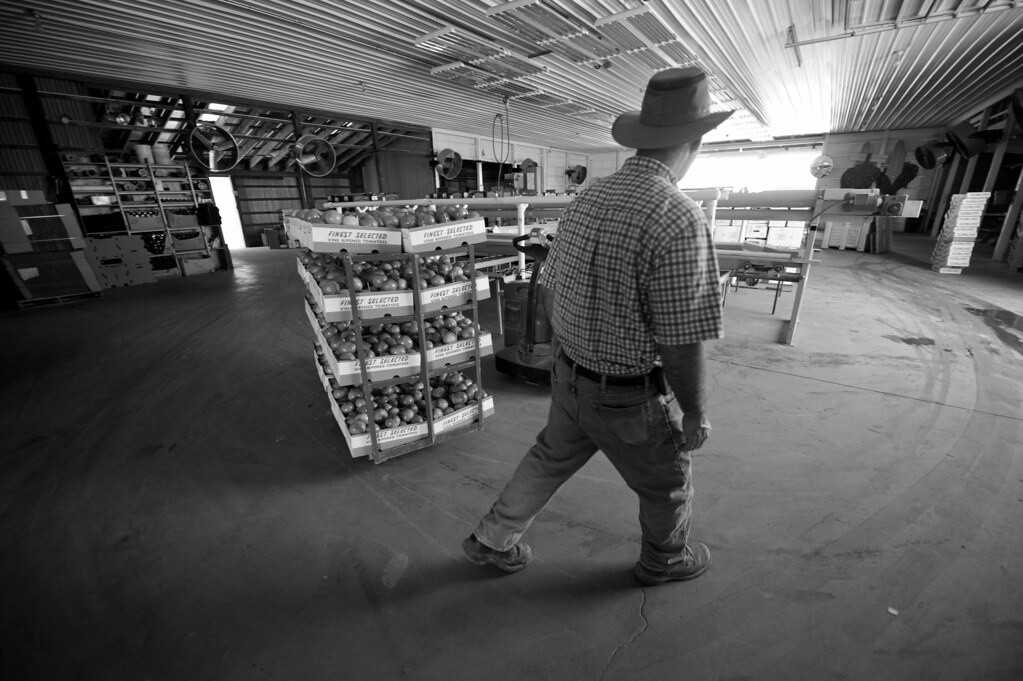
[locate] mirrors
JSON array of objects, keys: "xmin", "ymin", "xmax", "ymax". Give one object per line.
[{"xmin": 808, "ymin": 153, "xmax": 834, "ymax": 179}]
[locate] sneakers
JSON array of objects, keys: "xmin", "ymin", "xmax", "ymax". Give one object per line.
[
  {"xmin": 634, "ymin": 542, "xmax": 712, "ymax": 585},
  {"xmin": 462, "ymin": 533, "xmax": 532, "ymax": 572}
]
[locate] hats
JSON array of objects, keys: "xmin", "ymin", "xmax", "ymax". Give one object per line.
[{"xmin": 611, "ymin": 67, "xmax": 736, "ymax": 149}]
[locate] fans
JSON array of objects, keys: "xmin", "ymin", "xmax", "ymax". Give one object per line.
[
  {"xmin": 189, "ymin": 124, "xmax": 240, "ymax": 173},
  {"xmin": 566, "ymin": 165, "xmax": 587, "ymax": 185},
  {"xmin": 915, "ymin": 138, "xmax": 955, "ymax": 169},
  {"xmin": 292, "ymin": 134, "xmax": 336, "ymax": 178},
  {"xmin": 427, "ymin": 149, "xmax": 462, "ymax": 180},
  {"xmin": 942, "ymin": 120, "xmax": 1003, "ymax": 161}
]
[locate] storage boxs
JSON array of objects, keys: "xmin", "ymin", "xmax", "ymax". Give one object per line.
[
  {"xmin": 284, "ymin": 197, "xmax": 501, "ymax": 460},
  {"xmin": 123, "ymin": 207, "xmax": 216, "ymax": 275}
]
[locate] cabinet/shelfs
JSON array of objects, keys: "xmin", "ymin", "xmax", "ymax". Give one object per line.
[
  {"xmin": 326, "ymin": 245, "xmax": 484, "ymax": 466},
  {"xmin": 61, "ymin": 156, "xmax": 226, "ymax": 276}
]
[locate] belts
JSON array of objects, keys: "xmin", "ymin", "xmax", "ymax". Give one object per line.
[{"xmin": 561, "ymin": 348, "xmax": 657, "ymax": 386}]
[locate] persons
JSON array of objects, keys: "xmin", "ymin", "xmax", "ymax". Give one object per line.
[{"xmin": 459, "ymin": 67, "xmax": 737, "ymax": 585}]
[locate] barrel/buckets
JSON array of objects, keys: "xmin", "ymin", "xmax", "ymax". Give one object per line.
[
  {"xmin": 134, "ymin": 144, "xmax": 155, "ymax": 165},
  {"xmin": 152, "ymin": 144, "xmax": 171, "ymax": 164}
]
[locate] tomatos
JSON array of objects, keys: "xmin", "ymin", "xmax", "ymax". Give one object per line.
[{"xmin": 290, "ymin": 208, "xmax": 486, "ymax": 435}]
